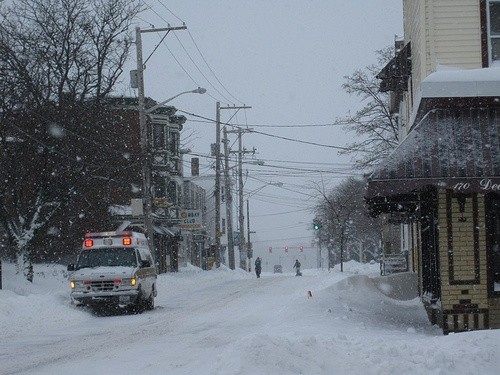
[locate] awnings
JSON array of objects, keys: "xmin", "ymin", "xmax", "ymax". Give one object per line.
[
  {"xmin": 363, "ymin": 108, "xmax": 500, "ymax": 219},
  {"xmin": 375, "ymin": 40, "xmax": 412, "ymax": 93}
]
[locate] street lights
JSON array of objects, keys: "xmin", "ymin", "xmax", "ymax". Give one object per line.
[
  {"xmin": 138, "ymin": 88, "xmax": 206, "ymax": 270},
  {"xmin": 215, "ymin": 160, "xmax": 265, "ymax": 268},
  {"xmin": 238, "ymin": 182, "xmax": 285, "ymax": 272}
]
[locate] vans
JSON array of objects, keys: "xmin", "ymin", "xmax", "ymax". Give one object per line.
[{"xmin": 66, "ymin": 232, "xmax": 158, "ymax": 315}]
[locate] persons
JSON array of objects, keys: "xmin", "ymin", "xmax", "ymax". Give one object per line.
[
  {"xmin": 293, "ymin": 259, "xmax": 301, "ymax": 274},
  {"xmin": 254, "ymin": 257, "xmax": 261, "ymax": 278}
]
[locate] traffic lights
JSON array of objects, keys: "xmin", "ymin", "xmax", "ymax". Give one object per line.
[
  {"xmin": 268, "ymin": 247, "xmax": 273, "ymax": 254},
  {"xmin": 284, "ymin": 245, "xmax": 288, "ymax": 252},
  {"xmin": 190, "ymin": 158, "xmax": 199, "ymax": 176},
  {"xmin": 299, "ymin": 245, "xmax": 304, "ymax": 252}
]
